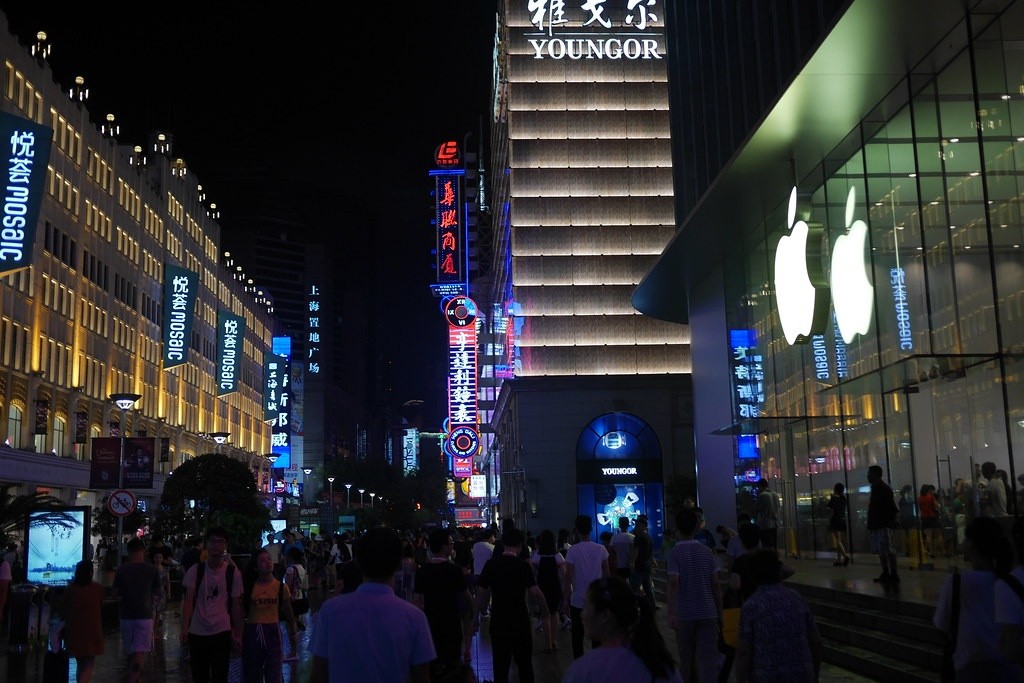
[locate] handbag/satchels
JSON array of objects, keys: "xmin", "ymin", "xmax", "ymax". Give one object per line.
[{"xmin": 938, "ymin": 634, "xmax": 959, "ymax": 682}]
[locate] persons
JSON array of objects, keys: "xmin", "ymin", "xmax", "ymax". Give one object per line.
[{"xmin": 3, "ymin": 447, "xmax": 1024, "ymax": 683}]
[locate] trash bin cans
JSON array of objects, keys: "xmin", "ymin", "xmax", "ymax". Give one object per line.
[
  {"xmin": 37, "ymin": 584, "xmax": 52, "ymax": 645},
  {"xmin": 7, "ymin": 584, "xmax": 40, "ymax": 649}
]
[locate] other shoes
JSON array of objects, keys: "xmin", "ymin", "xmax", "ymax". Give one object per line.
[
  {"xmin": 553, "ymin": 641, "xmax": 558, "ymax": 651},
  {"xmin": 833, "ymin": 562, "xmax": 841, "ymax": 567},
  {"xmin": 544, "ymin": 648, "xmax": 552, "ymax": 654},
  {"xmin": 843, "ymin": 556, "xmax": 849, "ymax": 565},
  {"xmin": 873, "ymin": 575, "xmax": 885, "ymax": 582},
  {"xmin": 283, "ymin": 654, "xmax": 299, "ymax": 662}
]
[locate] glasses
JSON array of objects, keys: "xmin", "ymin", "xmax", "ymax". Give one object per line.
[{"xmin": 207, "ymin": 539, "xmax": 224, "ymax": 545}]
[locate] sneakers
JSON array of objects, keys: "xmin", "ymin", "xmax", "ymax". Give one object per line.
[
  {"xmin": 559, "ymin": 618, "xmax": 572, "ymax": 629},
  {"xmin": 535, "ymin": 619, "xmax": 544, "ymax": 632}
]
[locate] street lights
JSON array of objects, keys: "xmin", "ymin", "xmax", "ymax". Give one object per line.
[
  {"xmin": 302, "ymin": 464, "xmax": 313, "ymax": 508},
  {"xmin": 369, "ymin": 492, "xmax": 376, "ymax": 507},
  {"xmin": 264, "ymin": 451, "xmax": 281, "ymax": 519},
  {"xmin": 379, "ymin": 495, "xmax": 383, "ymax": 504},
  {"xmin": 110, "ymin": 391, "xmax": 142, "ymax": 573},
  {"xmin": 345, "ymin": 483, "xmax": 352, "ymax": 508},
  {"xmin": 359, "ymin": 488, "xmax": 366, "ymax": 509},
  {"xmin": 210, "ymin": 431, "xmax": 230, "ymax": 454},
  {"xmin": 328, "ymin": 476, "xmax": 336, "ymax": 506}
]
